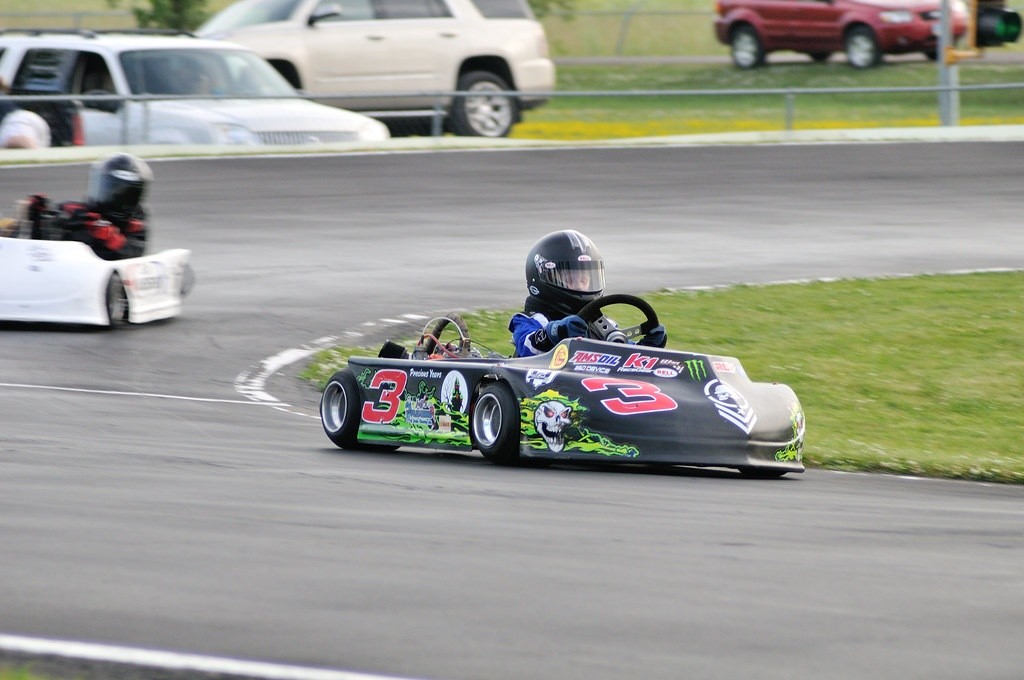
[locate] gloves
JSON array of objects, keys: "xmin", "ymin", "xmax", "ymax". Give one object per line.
[
  {"xmin": 546, "ymin": 315, "xmax": 588, "ymax": 345},
  {"xmin": 637, "ymin": 325, "xmax": 665, "ymax": 346},
  {"xmin": 86, "ymin": 220, "xmax": 126, "ymax": 251}
]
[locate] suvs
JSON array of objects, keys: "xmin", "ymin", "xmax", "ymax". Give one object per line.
[{"xmin": 0, "ymin": 12, "xmax": 390, "ymax": 150}]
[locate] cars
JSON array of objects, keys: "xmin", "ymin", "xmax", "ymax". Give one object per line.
[
  {"xmin": 714, "ymin": 0, "xmax": 968, "ymax": 70},
  {"xmin": 191, "ymin": 0, "xmax": 556, "ymax": 137}
]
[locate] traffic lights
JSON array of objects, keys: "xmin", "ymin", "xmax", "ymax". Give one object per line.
[{"xmin": 975, "ymin": 0, "xmax": 1022, "ymax": 49}]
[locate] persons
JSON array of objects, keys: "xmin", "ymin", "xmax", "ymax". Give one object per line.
[
  {"xmin": 508, "ymin": 229, "xmax": 665, "ymax": 357},
  {"xmin": 27, "ymin": 151, "xmax": 153, "ymax": 258}
]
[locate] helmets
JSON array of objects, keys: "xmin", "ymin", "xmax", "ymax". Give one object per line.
[
  {"xmin": 525, "ymin": 230, "xmax": 606, "ymax": 315},
  {"xmin": 99, "ymin": 154, "xmax": 144, "ymax": 213}
]
